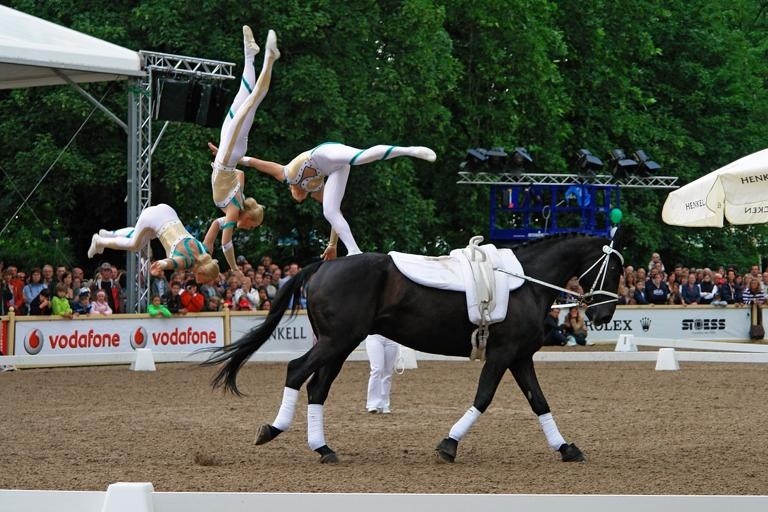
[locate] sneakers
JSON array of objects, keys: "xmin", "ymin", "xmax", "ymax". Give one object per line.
[
  {"xmin": 367, "ymin": 407, "xmax": 391, "ymax": 414},
  {"xmin": 242, "ymin": 25, "xmax": 280, "ymax": 59},
  {"xmin": 88, "ymin": 229, "xmax": 106, "ymax": 258},
  {"xmin": 414, "ymin": 146, "xmax": 437, "ymax": 162}
]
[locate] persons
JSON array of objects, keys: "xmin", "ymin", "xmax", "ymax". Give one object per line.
[
  {"xmin": 561, "ymin": 307, "xmax": 588, "ymax": 346},
  {"xmin": 1, "ymin": 252, "xmax": 768, "ymax": 316},
  {"xmin": 366, "ymin": 333, "xmax": 396, "ymax": 414},
  {"xmin": 202, "ymin": 26, "xmax": 280, "ymax": 280},
  {"xmin": 207, "ymin": 139, "xmax": 437, "ymax": 261},
  {"xmin": 550, "ymin": 306, "xmax": 567, "ymax": 345},
  {"xmin": 87, "ymin": 203, "xmax": 221, "ymax": 284}
]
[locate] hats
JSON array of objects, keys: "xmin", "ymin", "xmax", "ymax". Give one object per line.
[{"xmin": 102, "ymin": 262, "xmax": 112, "ymax": 269}]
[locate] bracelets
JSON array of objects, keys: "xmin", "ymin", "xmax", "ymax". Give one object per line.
[
  {"xmin": 241, "ymin": 156, "xmax": 251, "ymax": 166},
  {"xmin": 328, "ymin": 239, "xmax": 337, "ymax": 246}
]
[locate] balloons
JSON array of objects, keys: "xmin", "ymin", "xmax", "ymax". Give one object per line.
[{"xmin": 609, "ymin": 208, "xmax": 622, "ymax": 225}]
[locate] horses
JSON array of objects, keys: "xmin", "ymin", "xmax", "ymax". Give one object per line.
[{"xmin": 190, "ymin": 221, "xmax": 626, "ymax": 465}]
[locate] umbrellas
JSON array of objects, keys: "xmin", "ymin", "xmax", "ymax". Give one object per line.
[{"xmin": 662, "ymin": 147, "xmax": 768, "ymax": 228}]
